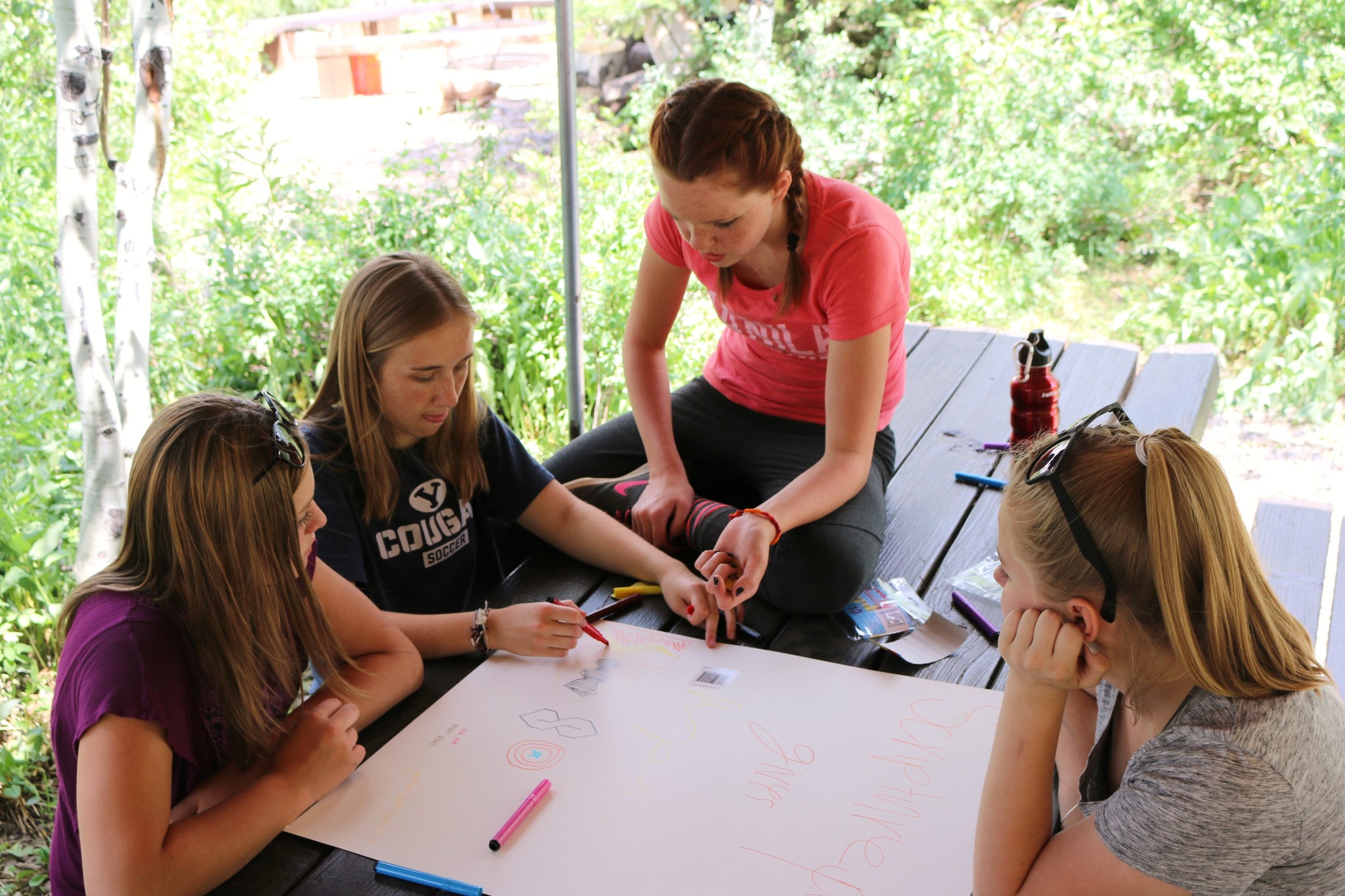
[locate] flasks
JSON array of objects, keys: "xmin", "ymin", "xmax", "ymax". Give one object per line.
[{"xmin": 1010, "ymin": 330, "xmax": 1058, "ymax": 450}]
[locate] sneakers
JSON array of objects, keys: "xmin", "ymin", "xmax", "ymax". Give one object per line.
[{"xmin": 545, "ymin": 461, "xmax": 651, "ymax": 533}]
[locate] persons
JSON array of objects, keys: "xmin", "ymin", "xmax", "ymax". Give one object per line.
[
  {"xmin": 278, "ymin": 255, "xmax": 745, "ymax": 713},
  {"xmin": 487, "ymin": 78, "xmax": 914, "ymax": 618},
  {"xmin": 35, "ymin": 392, "xmax": 424, "ymax": 895},
  {"xmin": 971, "ymin": 401, "xmax": 1345, "ymax": 896}
]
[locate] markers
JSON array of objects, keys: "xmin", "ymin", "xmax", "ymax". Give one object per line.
[
  {"xmin": 719, "ymin": 612, "xmax": 767, "ymax": 644},
  {"xmin": 489, "ymin": 779, "xmax": 552, "ymax": 852},
  {"xmin": 373, "ymin": 860, "xmax": 483, "ymax": 896},
  {"xmin": 584, "ymin": 593, "xmax": 643, "ymax": 624},
  {"xmin": 984, "ymin": 442, "xmax": 1011, "ymax": 451},
  {"xmin": 613, "ymin": 585, "xmax": 663, "ymax": 597},
  {"xmin": 955, "ymin": 471, "xmax": 1010, "ymax": 492},
  {"xmin": 546, "ymin": 596, "xmax": 610, "ymax": 646},
  {"xmin": 952, "ymin": 590, "xmax": 1000, "ymax": 642}
]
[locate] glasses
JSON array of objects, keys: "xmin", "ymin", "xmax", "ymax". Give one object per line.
[
  {"xmin": 235, "ymin": 386, "xmax": 309, "ymax": 495},
  {"xmin": 1023, "ymin": 390, "xmax": 1148, "ymax": 626}
]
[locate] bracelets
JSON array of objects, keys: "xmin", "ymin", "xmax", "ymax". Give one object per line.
[
  {"xmin": 723, "ymin": 508, "xmax": 784, "ymax": 545},
  {"xmin": 468, "ymin": 607, "xmax": 493, "ymax": 656}
]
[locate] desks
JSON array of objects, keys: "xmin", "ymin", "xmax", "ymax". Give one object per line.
[{"xmin": 208, "ymin": 320, "xmax": 1226, "ymax": 896}]
